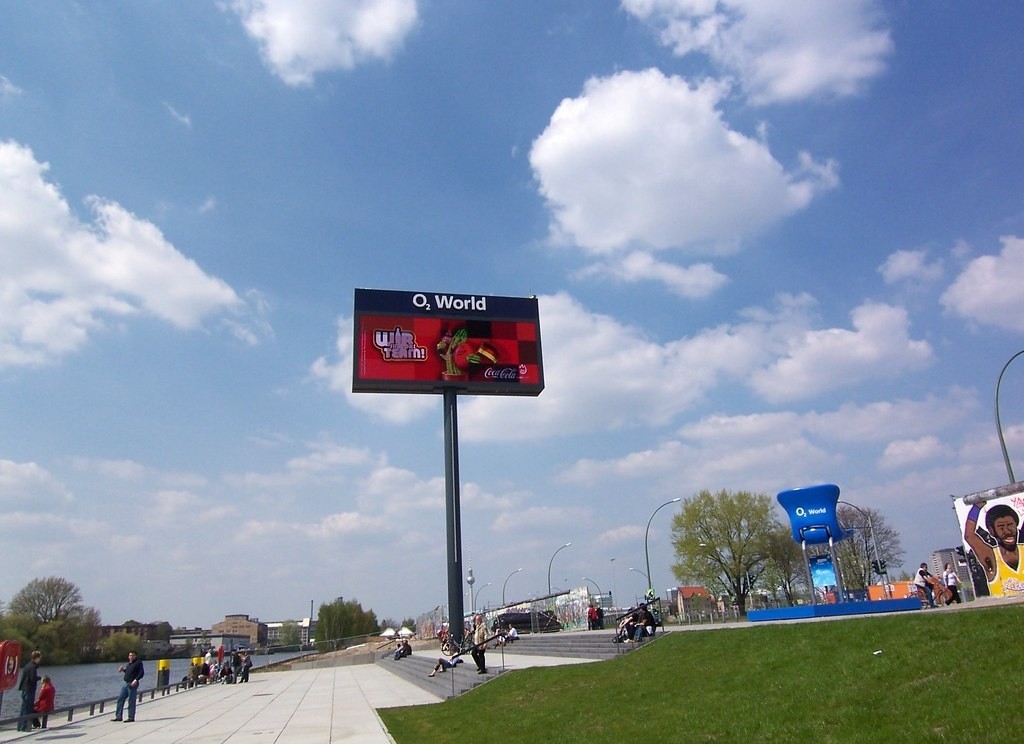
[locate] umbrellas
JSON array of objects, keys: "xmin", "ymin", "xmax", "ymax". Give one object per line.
[{"xmin": 217, "ymin": 646, "xmax": 224, "ymax": 669}]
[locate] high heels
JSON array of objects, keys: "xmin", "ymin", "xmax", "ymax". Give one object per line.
[{"xmin": 32, "ymin": 724, "xmax": 41, "ymax": 729}]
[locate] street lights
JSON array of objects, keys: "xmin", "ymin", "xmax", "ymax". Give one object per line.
[
  {"xmin": 502, "ymin": 567, "xmax": 525, "ymax": 606},
  {"xmin": 836, "ymin": 500, "xmax": 887, "ymax": 600},
  {"xmin": 583, "ymin": 577, "xmax": 603, "ymax": 610},
  {"xmin": 645, "ymin": 497, "xmax": 682, "ymax": 590},
  {"xmin": 474, "ymin": 582, "xmax": 492, "ymax": 614},
  {"xmin": 548, "ymin": 543, "xmax": 572, "ymax": 594}
]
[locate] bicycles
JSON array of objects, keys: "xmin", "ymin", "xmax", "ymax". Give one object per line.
[
  {"xmin": 441, "ymin": 633, "xmax": 474, "ymax": 656},
  {"xmin": 908, "ymin": 580, "xmax": 951, "ymax": 610}
]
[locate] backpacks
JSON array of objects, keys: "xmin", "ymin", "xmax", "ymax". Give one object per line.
[{"xmin": 612, "ymin": 630, "xmax": 628, "ymax": 643}]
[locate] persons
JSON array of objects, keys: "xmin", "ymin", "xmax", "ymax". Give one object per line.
[
  {"xmin": 31, "ymin": 675, "xmax": 55, "ymax": 729},
  {"xmin": 394, "ymin": 640, "xmax": 412, "ymax": 658},
  {"xmin": 427, "ymin": 646, "xmax": 463, "ymax": 677},
  {"xmin": 618, "ymin": 605, "xmax": 656, "ymax": 642},
  {"xmin": 205, "ymin": 649, "xmax": 212, "ymax": 666},
  {"xmin": 188, "ymin": 659, "xmax": 233, "ymax": 685},
  {"xmin": 493, "ymin": 624, "xmax": 519, "ymax": 649},
  {"xmin": 942, "ymin": 563, "xmax": 962, "ymax": 608},
  {"xmin": 111, "ymin": 651, "xmax": 144, "ymax": 723},
  {"xmin": 438, "ymin": 624, "xmax": 450, "ymax": 650},
  {"xmin": 231, "ymin": 649, "xmax": 253, "ymax": 684},
  {"xmin": 17, "ymin": 651, "xmax": 42, "ymax": 731},
  {"xmin": 463, "ymin": 628, "xmax": 472, "ymax": 647},
  {"xmin": 587, "ymin": 605, "xmax": 598, "ymax": 631},
  {"xmin": 595, "ymin": 604, "xmax": 604, "ymax": 630},
  {"xmin": 732, "ymin": 602, "xmax": 740, "ymax": 619},
  {"xmin": 471, "ymin": 615, "xmax": 488, "ymax": 674},
  {"xmin": 914, "ymin": 562, "xmax": 939, "ymax": 608}
]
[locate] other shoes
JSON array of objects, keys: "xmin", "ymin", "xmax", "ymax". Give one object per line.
[
  {"xmin": 623, "ymin": 638, "xmax": 631, "ymax": 643},
  {"xmin": 944, "ymin": 601, "xmax": 949, "ymax": 607},
  {"xmin": 931, "ymin": 605, "xmax": 939, "ymax": 608},
  {"xmin": 428, "ymin": 675, "xmax": 435, "ymax": 677},
  {"xmin": 477, "ymin": 667, "xmax": 481, "ymax": 671},
  {"xmin": 630, "ymin": 639, "xmax": 641, "ymax": 642},
  {"xmin": 110, "ymin": 718, "xmax": 122, "ymax": 721},
  {"xmin": 478, "ymin": 671, "xmax": 487, "ymax": 674},
  {"xmin": 123, "ymin": 718, "xmax": 135, "ymax": 722},
  {"xmin": 17, "ymin": 726, "xmax": 34, "ymax": 732}
]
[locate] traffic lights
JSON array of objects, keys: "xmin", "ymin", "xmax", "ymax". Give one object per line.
[
  {"xmin": 955, "ymin": 545, "xmax": 967, "ymax": 563},
  {"xmin": 880, "ymin": 559, "xmax": 886, "ymax": 569},
  {"xmin": 872, "ymin": 560, "xmax": 879, "ymax": 574}
]
